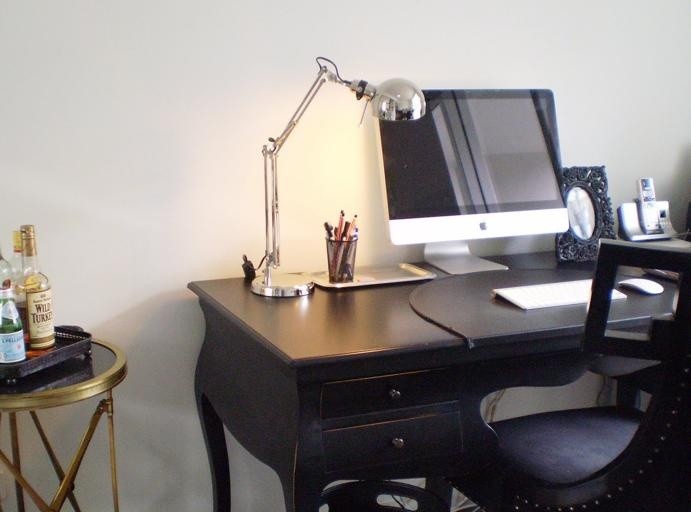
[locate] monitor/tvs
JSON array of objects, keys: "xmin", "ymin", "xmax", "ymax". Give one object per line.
[{"xmin": 378, "ymin": 87, "xmax": 569, "ymax": 274}]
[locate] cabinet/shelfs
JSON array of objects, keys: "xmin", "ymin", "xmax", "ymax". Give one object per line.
[{"xmin": 186, "ymin": 232, "xmax": 691, "ymax": 512}]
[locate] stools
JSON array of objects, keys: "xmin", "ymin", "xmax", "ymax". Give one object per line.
[{"xmin": 0, "ymin": 335, "xmax": 129, "ymax": 511}]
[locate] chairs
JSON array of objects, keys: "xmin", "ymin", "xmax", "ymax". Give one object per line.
[{"xmin": 423, "ymin": 239, "xmax": 690, "ymax": 512}]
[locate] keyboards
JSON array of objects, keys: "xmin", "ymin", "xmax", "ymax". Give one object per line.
[{"xmin": 491, "ymin": 279, "xmax": 627, "ymax": 312}]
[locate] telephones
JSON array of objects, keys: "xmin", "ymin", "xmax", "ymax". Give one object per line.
[{"xmin": 619, "ymin": 177, "xmax": 677, "ymax": 241}]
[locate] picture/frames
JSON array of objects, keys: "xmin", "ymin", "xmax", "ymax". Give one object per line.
[{"xmin": 554, "ymin": 165, "xmax": 617, "ymax": 267}]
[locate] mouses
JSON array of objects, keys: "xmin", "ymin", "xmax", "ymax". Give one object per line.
[{"xmin": 619, "ymin": 278, "xmax": 664, "ymax": 295}]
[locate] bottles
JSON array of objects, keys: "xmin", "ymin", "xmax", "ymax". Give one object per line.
[
  {"xmin": 0, "ymin": 252, "xmax": 11, "ymax": 277},
  {"xmin": 10, "ymin": 232, "xmax": 28, "ymax": 280},
  {"xmin": 19, "ymin": 225, "xmax": 56, "ymax": 348},
  {"xmin": 0, "ymin": 278, "xmax": 28, "ymax": 363}
]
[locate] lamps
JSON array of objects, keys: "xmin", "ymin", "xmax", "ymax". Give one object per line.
[{"xmin": 249, "ymin": 53, "xmax": 428, "ymax": 299}]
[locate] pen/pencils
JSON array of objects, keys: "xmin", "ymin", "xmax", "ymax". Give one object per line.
[
  {"xmin": 400, "ymin": 263, "xmax": 437, "ymax": 277},
  {"xmin": 324, "ymin": 210, "xmax": 358, "ymax": 282}
]
